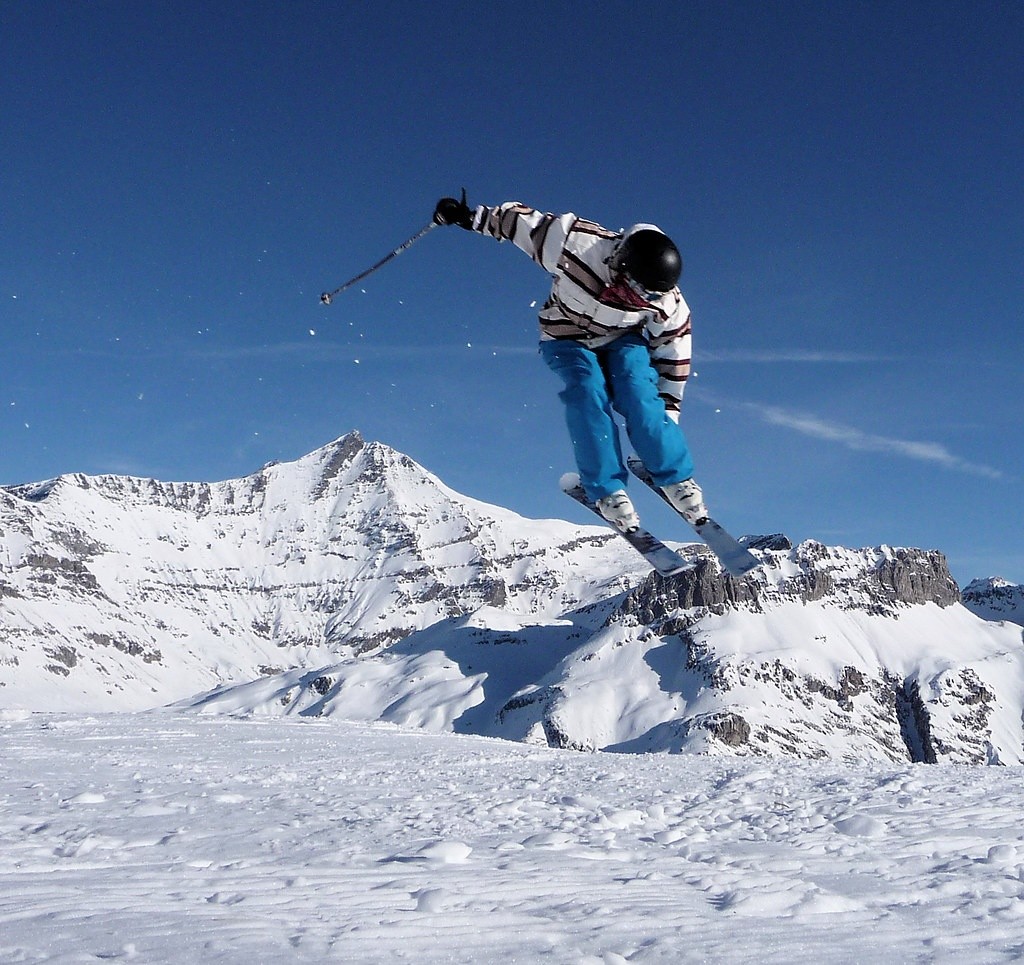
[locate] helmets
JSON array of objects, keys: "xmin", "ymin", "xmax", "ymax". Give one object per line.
[{"xmin": 624, "ymin": 229, "xmax": 682, "ymax": 292}]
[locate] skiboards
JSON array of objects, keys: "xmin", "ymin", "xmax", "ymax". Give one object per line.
[
  {"xmin": 627, "ymin": 452, "xmax": 762, "ymax": 579},
  {"xmin": 559, "ymin": 471, "xmax": 693, "ymax": 576}
]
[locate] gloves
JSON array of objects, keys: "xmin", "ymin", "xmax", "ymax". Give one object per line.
[{"xmin": 433, "ymin": 198, "xmax": 477, "ymax": 231}]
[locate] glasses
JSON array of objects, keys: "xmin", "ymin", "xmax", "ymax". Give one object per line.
[{"xmin": 623, "ymin": 271, "xmax": 675, "ymax": 301}]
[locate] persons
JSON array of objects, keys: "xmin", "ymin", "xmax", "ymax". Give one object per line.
[{"xmin": 432, "ymin": 188, "xmax": 708, "ymax": 533}]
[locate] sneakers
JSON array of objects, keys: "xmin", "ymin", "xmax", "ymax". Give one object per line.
[
  {"xmin": 661, "ymin": 477, "xmax": 703, "ymax": 513},
  {"xmin": 596, "ymin": 489, "xmax": 634, "ymax": 521}
]
